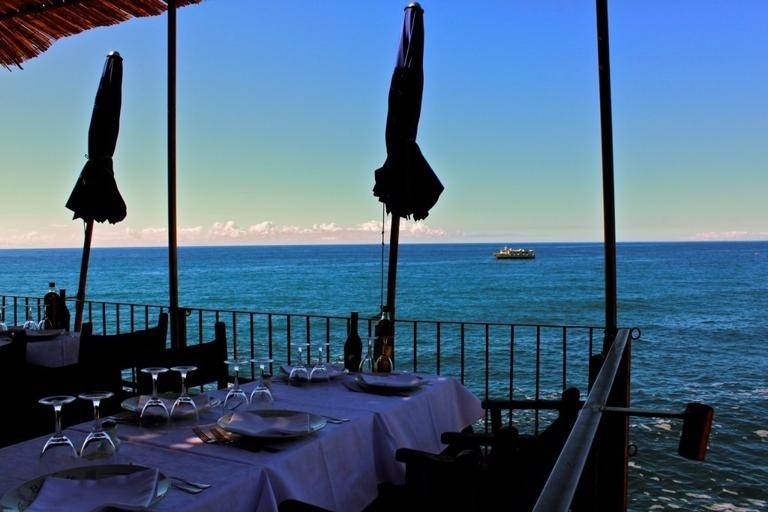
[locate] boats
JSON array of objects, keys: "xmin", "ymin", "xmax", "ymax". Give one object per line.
[{"xmin": 493, "ymin": 246, "xmax": 535, "ymax": 258}]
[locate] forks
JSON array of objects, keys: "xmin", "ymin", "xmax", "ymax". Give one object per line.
[
  {"xmin": 342, "ymin": 381, "xmax": 409, "ymax": 398},
  {"xmin": 192, "ymin": 427, "xmax": 280, "ymax": 454}
]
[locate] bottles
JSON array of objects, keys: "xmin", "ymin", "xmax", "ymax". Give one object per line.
[
  {"xmin": 375, "ymin": 305, "xmax": 394, "ymax": 360},
  {"xmin": 44, "ymin": 282, "xmax": 70, "ymax": 331},
  {"xmin": 344, "ymin": 311, "xmax": 362, "ymax": 369}
]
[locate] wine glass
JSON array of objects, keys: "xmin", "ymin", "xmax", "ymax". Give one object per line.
[
  {"xmin": 289, "ymin": 342, "xmax": 330, "ymax": 385},
  {"xmin": 24, "ymin": 304, "xmax": 44, "ymax": 329},
  {"xmin": 37, "ymin": 390, "xmax": 117, "ymax": 463},
  {"xmin": 0, "ymin": 306, "xmax": 7, "ymax": 331},
  {"xmin": 360, "ymin": 336, "xmax": 394, "ymax": 372},
  {"xmin": 140, "ymin": 366, "xmax": 199, "ymax": 429},
  {"xmin": 223, "ymin": 358, "xmax": 274, "ymax": 408}
]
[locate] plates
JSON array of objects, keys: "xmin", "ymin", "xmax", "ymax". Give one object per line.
[
  {"xmin": 362, "ymin": 374, "xmax": 424, "ymax": 388},
  {"xmin": 0, "ymin": 464, "xmax": 170, "ymax": 512},
  {"xmin": 10, "ymin": 329, "xmax": 64, "ymax": 341},
  {"xmin": 282, "ymin": 364, "xmax": 349, "ymax": 380},
  {"xmin": 121, "ymin": 393, "xmax": 220, "ymax": 415},
  {"xmin": 218, "ymin": 408, "xmax": 327, "ymax": 438}
]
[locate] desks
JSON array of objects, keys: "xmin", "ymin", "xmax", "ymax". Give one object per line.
[
  {"xmin": 2, "ymin": 326, "xmax": 82, "ymax": 366},
  {"xmin": 2, "ymin": 357, "xmax": 483, "ymax": 511}
]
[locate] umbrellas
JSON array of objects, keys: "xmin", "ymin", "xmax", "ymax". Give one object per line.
[
  {"xmin": 66, "ymin": 51, "xmax": 127, "ymax": 334},
  {"xmin": 372, "ymin": 2, "xmax": 445, "ymax": 370}
]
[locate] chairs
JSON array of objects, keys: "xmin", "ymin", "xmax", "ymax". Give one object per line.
[
  {"xmin": 155, "ymin": 321, "xmax": 230, "ymax": 394},
  {"xmin": 77, "ymin": 314, "xmax": 167, "ymax": 394},
  {"xmin": 440, "ymin": 386, "xmax": 580, "ymax": 474},
  {"xmin": 0, "ymin": 330, "xmax": 29, "ymax": 360},
  {"xmin": 279, "ymin": 445, "xmax": 542, "ymax": 511},
  {"xmin": 1, "ymin": 362, "xmax": 112, "ymax": 446}
]
